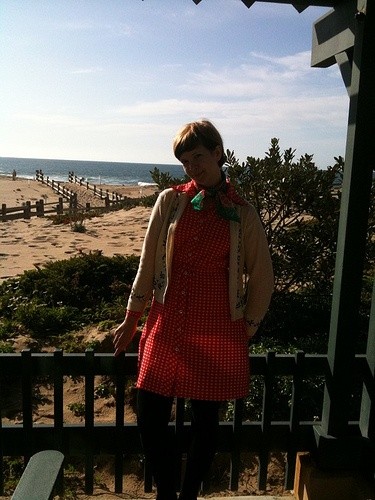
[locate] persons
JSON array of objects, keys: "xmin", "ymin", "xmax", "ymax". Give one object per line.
[
  {"xmin": 113, "ymin": 121, "xmax": 276, "ymax": 500},
  {"xmin": 12, "ymin": 169, "xmax": 17, "ymax": 181}
]
